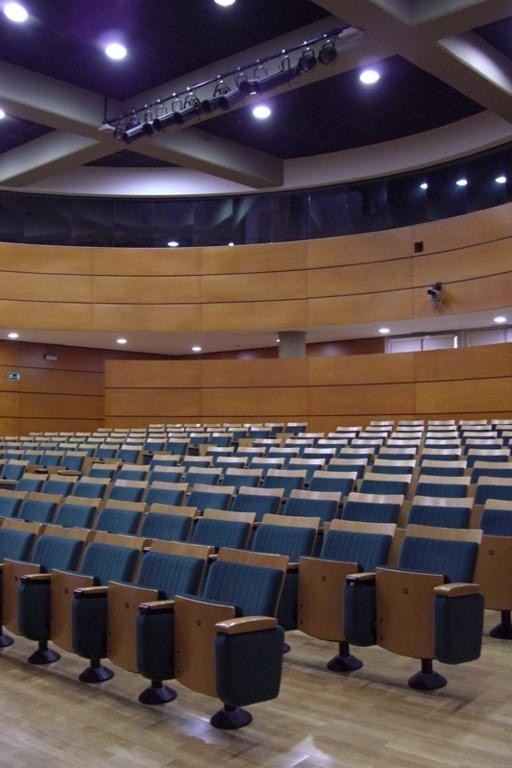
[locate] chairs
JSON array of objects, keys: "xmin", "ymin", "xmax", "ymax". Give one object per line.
[
  {"xmin": 0, "ymin": 418, "xmax": 512, "ymax": 691},
  {"xmin": 2, "ymin": 517, "xmax": 293, "ymax": 730}
]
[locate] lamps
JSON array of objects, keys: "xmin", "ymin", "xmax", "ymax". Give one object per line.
[{"xmin": 100, "ymin": 26, "xmax": 363, "ymax": 148}]
